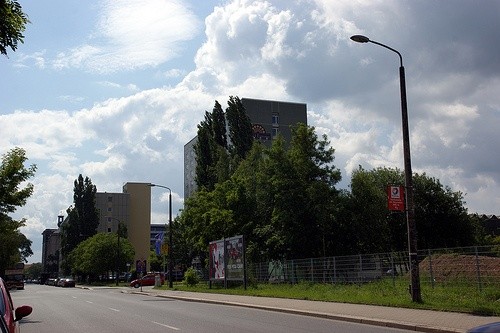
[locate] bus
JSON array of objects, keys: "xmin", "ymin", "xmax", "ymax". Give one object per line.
[{"xmin": 4, "ymin": 262, "xmax": 24, "ymax": 289}]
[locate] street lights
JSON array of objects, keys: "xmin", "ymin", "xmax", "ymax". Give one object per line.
[
  {"xmin": 350, "ymin": 34, "xmax": 423, "ymax": 304},
  {"xmin": 104, "ymin": 215, "xmax": 120, "ymax": 286},
  {"xmin": 148, "ymin": 183, "xmax": 173, "ymax": 287}
]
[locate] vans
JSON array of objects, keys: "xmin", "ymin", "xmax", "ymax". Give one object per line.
[{"xmin": 117, "ymin": 271, "xmax": 126, "ymax": 279}]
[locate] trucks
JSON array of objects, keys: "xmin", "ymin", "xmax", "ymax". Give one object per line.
[{"xmin": 38, "ymin": 272, "xmax": 51, "ymax": 285}]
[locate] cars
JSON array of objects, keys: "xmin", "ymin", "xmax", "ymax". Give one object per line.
[
  {"xmin": 109, "ymin": 275, "xmax": 117, "ymax": 280},
  {"xmin": 26, "ymin": 278, "xmax": 32, "ymax": 283},
  {"xmin": 32, "ymin": 279, "xmax": 38, "ymax": 284},
  {"xmin": 46, "ymin": 278, "xmax": 54, "ymax": 286},
  {"xmin": 130, "ymin": 272, "xmax": 164, "ymax": 288},
  {"xmin": 126, "ymin": 274, "xmax": 132, "ymax": 281},
  {"xmin": 98, "ymin": 275, "xmax": 106, "ymax": 280},
  {"xmin": 54, "ymin": 277, "xmax": 77, "ymax": 287},
  {"xmin": 0, "ymin": 277, "xmax": 33, "ymax": 333},
  {"xmin": 23, "ymin": 280, "xmax": 27, "ymax": 283}
]
